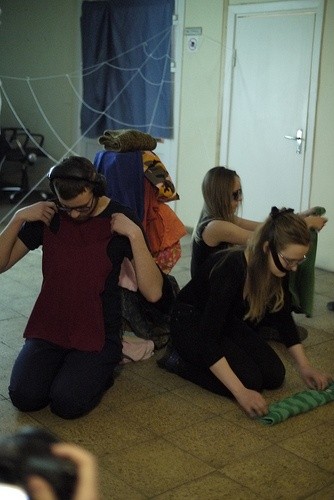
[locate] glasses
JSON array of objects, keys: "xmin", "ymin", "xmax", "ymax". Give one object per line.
[
  {"xmin": 56, "ymin": 195, "xmax": 94, "ymax": 212},
  {"xmin": 279, "ymin": 252, "xmax": 307, "ymax": 266},
  {"xmin": 232, "ymin": 189, "xmax": 241, "ymax": 202}
]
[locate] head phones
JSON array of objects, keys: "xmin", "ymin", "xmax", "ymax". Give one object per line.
[{"xmin": 49, "ymin": 163, "xmax": 106, "ymax": 198}]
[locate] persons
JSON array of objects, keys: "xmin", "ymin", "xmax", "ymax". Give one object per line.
[
  {"xmin": 0, "ymin": 156, "xmax": 164, "ymax": 419},
  {"xmin": 191, "ymin": 167, "xmax": 328, "ymax": 280}
]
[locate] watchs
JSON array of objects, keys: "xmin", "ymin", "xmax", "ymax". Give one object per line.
[{"xmin": 156, "ymin": 205, "xmax": 329, "ymax": 418}]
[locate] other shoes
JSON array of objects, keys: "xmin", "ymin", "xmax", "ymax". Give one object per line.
[{"xmin": 156, "ymin": 344, "xmax": 183, "ymax": 371}]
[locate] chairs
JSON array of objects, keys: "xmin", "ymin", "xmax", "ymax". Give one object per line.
[{"xmin": 0, "ymin": 127, "xmax": 51, "ymax": 202}]
